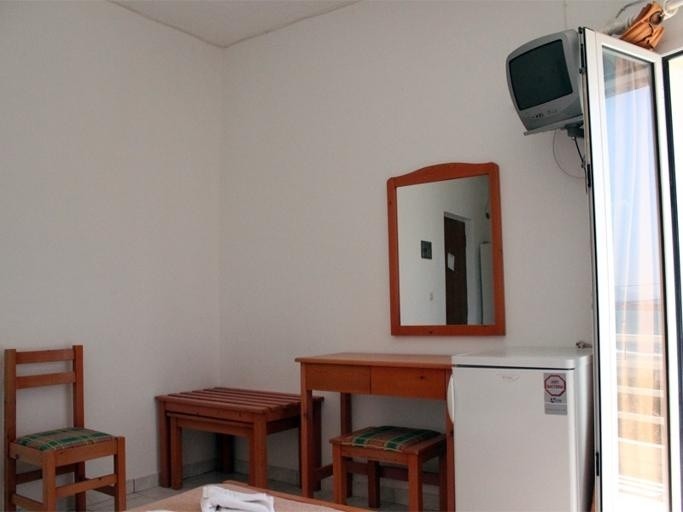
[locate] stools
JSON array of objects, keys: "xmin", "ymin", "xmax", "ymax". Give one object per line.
[{"xmin": 326, "ymin": 423, "xmax": 448, "ymax": 511}]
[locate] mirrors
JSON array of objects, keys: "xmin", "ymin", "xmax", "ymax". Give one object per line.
[{"xmin": 385, "ymin": 160, "xmax": 507, "ymax": 338}]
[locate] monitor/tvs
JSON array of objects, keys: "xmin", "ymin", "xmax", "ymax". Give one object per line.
[{"xmin": 505, "ymin": 29, "xmax": 584, "ymax": 136}]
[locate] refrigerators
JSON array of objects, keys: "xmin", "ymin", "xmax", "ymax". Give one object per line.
[{"xmin": 445, "ymin": 346, "xmax": 591, "ymax": 508}]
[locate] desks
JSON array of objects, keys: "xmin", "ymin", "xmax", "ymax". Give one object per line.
[
  {"xmin": 116, "ymin": 477, "xmax": 383, "ymax": 512},
  {"xmin": 154, "ymin": 385, "xmax": 325, "ymax": 494},
  {"xmin": 293, "ymin": 350, "xmax": 459, "ymax": 512}
]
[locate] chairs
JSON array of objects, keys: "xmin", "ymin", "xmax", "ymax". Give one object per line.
[{"xmin": 2, "ymin": 342, "xmax": 128, "ymax": 512}]
